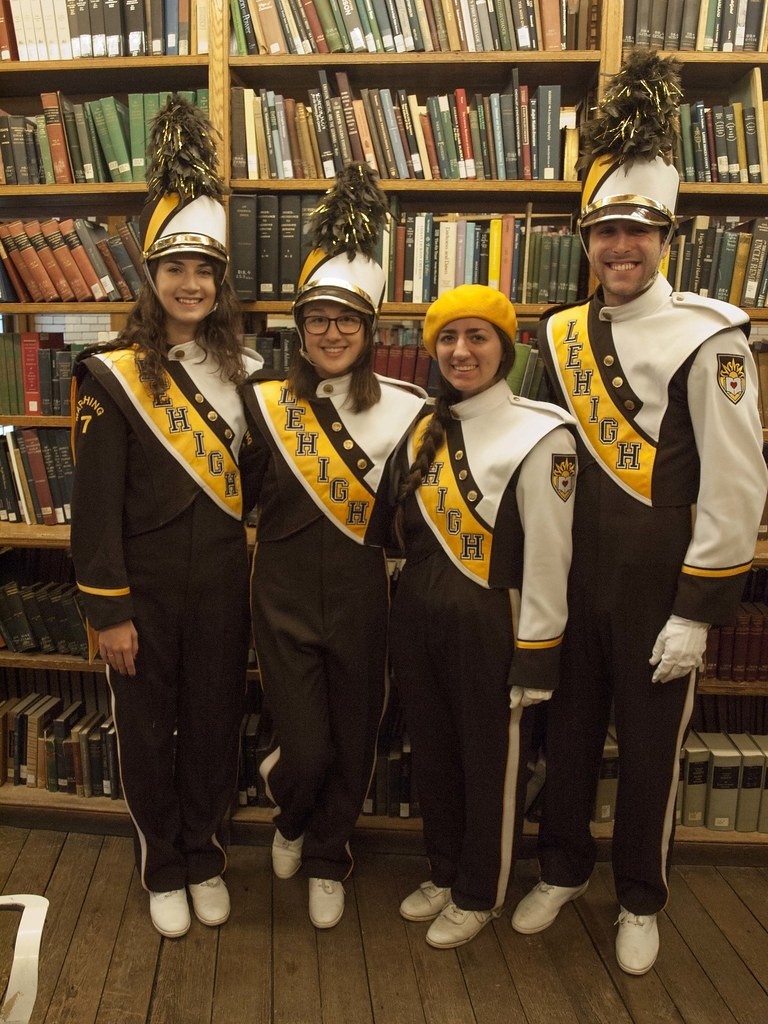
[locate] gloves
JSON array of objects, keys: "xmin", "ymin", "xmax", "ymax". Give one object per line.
[
  {"xmin": 510, "ymin": 684, "xmax": 554, "ymax": 708},
  {"xmin": 649, "ymin": 613, "xmax": 712, "ymax": 683}
]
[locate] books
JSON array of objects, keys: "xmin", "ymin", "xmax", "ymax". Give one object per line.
[{"xmin": 0, "ymin": 0, "xmax": 768, "ymax": 835}]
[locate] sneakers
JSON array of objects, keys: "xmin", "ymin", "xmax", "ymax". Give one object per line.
[
  {"xmin": 148, "ymin": 887, "xmax": 191, "ymax": 938},
  {"xmin": 309, "ymin": 878, "xmax": 344, "ymax": 928},
  {"xmin": 616, "ymin": 905, "xmax": 659, "ymax": 974},
  {"xmin": 272, "ymin": 827, "xmax": 303, "ymax": 879},
  {"xmin": 400, "ymin": 880, "xmax": 452, "ymax": 921},
  {"xmin": 190, "ymin": 875, "xmax": 230, "ymax": 925},
  {"xmin": 512, "ymin": 877, "xmax": 589, "ymax": 934},
  {"xmin": 426, "ymin": 899, "xmax": 502, "ymax": 948}
]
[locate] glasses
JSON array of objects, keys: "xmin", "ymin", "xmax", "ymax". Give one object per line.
[{"xmin": 299, "ymin": 313, "xmax": 365, "ymax": 335}]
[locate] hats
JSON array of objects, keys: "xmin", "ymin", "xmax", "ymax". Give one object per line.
[
  {"xmin": 293, "ymin": 159, "xmax": 386, "ymax": 336},
  {"xmin": 423, "ymin": 285, "xmax": 518, "ymax": 361},
  {"xmin": 576, "ymin": 50, "xmax": 680, "ymax": 244},
  {"xmin": 139, "ymin": 93, "xmax": 230, "ymax": 286}
]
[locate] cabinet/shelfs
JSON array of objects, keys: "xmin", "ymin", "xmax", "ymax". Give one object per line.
[{"xmin": 0, "ymin": 0, "xmax": 768, "ymax": 846}]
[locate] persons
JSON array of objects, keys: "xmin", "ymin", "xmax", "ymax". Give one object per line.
[
  {"xmin": 71, "ymin": 189, "xmax": 266, "ymax": 939},
  {"xmin": 405, "ymin": 284, "xmax": 576, "ymax": 948},
  {"xmin": 517, "ymin": 150, "xmax": 768, "ymax": 975},
  {"xmin": 238, "ymin": 250, "xmax": 431, "ymax": 931}
]
